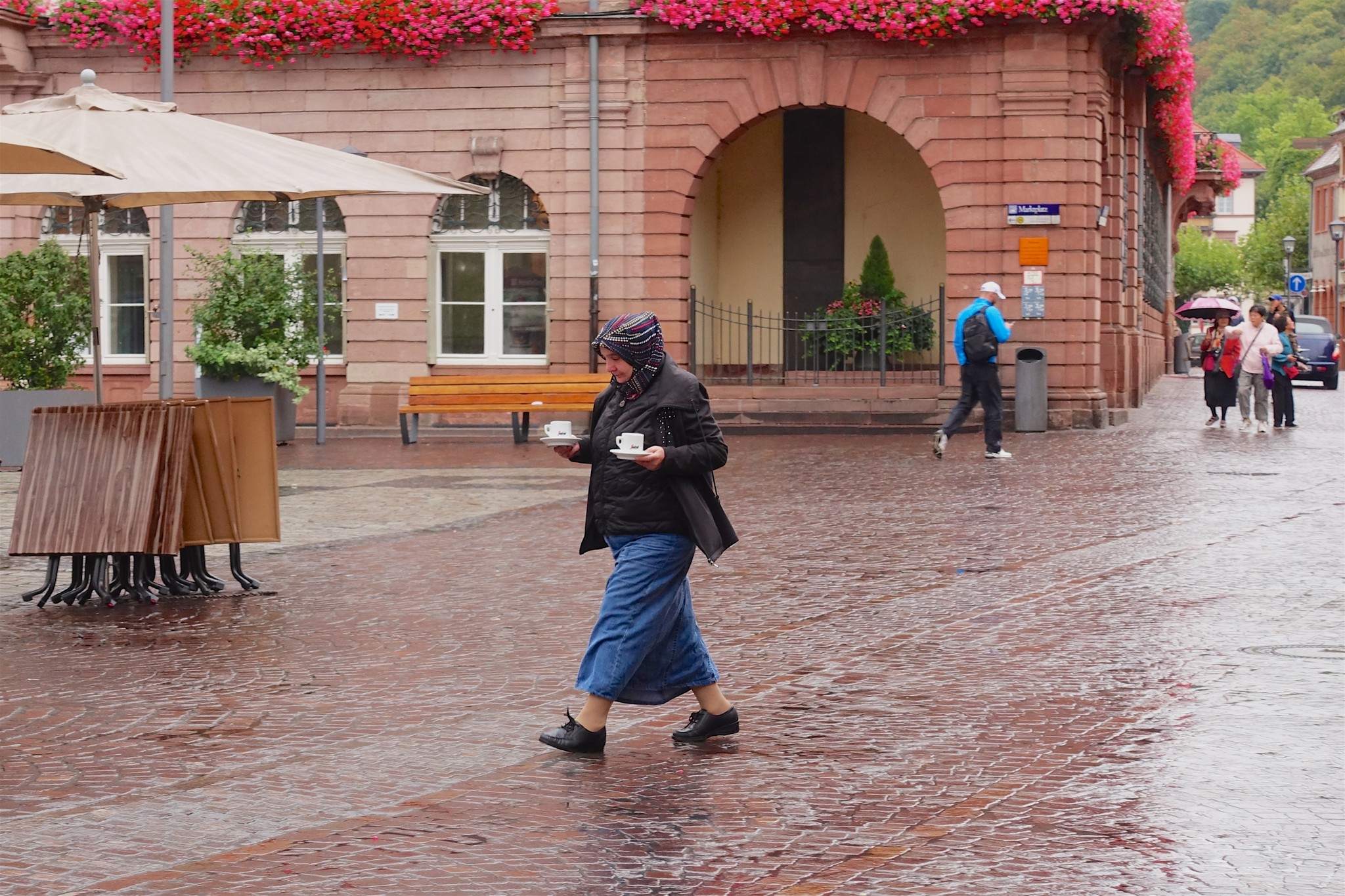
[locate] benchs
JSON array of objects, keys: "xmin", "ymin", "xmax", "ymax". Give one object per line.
[{"xmin": 400, "ymin": 374, "xmax": 615, "ymax": 447}]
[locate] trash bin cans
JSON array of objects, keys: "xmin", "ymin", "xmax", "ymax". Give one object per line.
[
  {"xmin": 1174, "ymin": 335, "xmax": 1190, "ymax": 374},
  {"xmin": 1015, "ymin": 346, "xmax": 1047, "ymax": 433}
]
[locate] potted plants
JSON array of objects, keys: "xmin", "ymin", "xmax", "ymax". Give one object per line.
[
  {"xmin": 0, "ymin": 236, "xmax": 98, "ymax": 471},
  {"xmin": 179, "ymin": 217, "xmax": 344, "ymax": 452},
  {"xmin": 793, "ymin": 235, "xmax": 937, "ymax": 371}
]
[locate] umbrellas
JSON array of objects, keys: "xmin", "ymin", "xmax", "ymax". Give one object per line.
[
  {"xmin": 2, "ymin": 67, "xmax": 491, "ymax": 404},
  {"xmin": 1175, "ymin": 296, "xmax": 1244, "ymax": 327}
]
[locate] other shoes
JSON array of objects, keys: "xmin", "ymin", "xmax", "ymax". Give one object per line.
[
  {"xmin": 1221, "ymin": 419, "xmax": 1227, "ymax": 427},
  {"xmin": 1273, "ymin": 424, "xmax": 1284, "ymax": 430},
  {"xmin": 1206, "ymin": 415, "xmax": 1219, "ymax": 425},
  {"xmin": 1286, "ymin": 423, "xmax": 1300, "ymax": 429}
]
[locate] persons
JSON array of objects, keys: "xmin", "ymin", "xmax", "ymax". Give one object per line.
[
  {"xmin": 1270, "ymin": 314, "xmax": 1311, "ymax": 431},
  {"xmin": 1224, "ymin": 305, "xmax": 1283, "ymax": 433},
  {"xmin": 1264, "ymin": 295, "xmax": 1296, "ymax": 330},
  {"xmin": 931, "ymin": 281, "xmax": 1016, "ymax": 459},
  {"xmin": 1200, "ymin": 309, "xmax": 1242, "ymax": 428},
  {"xmin": 538, "ymin": 310, "xmax": 744, "ymax": 756},
  {"xmin": 1271, "ymin": 312, "xmax": 1298, "ymax": 352}
]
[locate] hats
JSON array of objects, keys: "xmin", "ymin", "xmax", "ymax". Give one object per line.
[
  {"xmin": 980, "ymin": 281, "xmax": 1006, "ymax": 300},
  {"xmin": 1269, "ymin": 294, "xmax": 1282, "ymax": 301},
  {"xmin": 1213, "ymin": 310, "xmax": 1232, "ymax": 324}
]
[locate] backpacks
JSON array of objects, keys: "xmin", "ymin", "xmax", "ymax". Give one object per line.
[{"xmin": 962, "ymin": 306, "xmax": 998, "ymax": 365}]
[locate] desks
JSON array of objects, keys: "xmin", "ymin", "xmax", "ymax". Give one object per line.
[{"xmin": 6, "ymin": 391, "xmax": 293, "ymax": 612}]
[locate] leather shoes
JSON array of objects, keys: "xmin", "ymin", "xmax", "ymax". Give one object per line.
[
  {"xmin": 540, "ymin": 721, "xmax": 607, "ymax": 754},
  {"xmin": 672, "ymin": 705, "xmax": 739, "ymax": 742}
]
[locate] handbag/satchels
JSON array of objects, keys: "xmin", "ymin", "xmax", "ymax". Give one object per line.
[
  {"xmin": 1202, "ymin": 353, "xmax": 1214, "ymax": 371},
  {"xmin": 1262, "ymin": 353, "xmax": 1275, "ymax": 390},
  {"xmin": 1233, "ymin": 363, "xmax": 1242, "ymax": 380},
  {"xmin": 1284, "ymin": 363, "xmax": 1299, "ymax": 379}
]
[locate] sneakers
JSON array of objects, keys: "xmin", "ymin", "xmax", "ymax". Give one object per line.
[
  {"xmin": 985, "ymin": 448, "xmax": 1011, "ymax": 458},
  {"xmin": 1238, "ymin": 418, "xmax": 1252, "ymax": 430},
  {"xmin": 1257, "ymin": 422, "xmax": 1267, "ymax": 433},
  {"xmin": 933, "ymin": 429, "xmax": 947, "ymax": 458}
]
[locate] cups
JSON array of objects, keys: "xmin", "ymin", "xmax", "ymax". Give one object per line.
[
  {"xmin": 544, "ymin": 420, "xmax": 572, "ymax": 438},
  {"xmin": 616, "ymin": 432, "xmax": 645, "ymax": 454}
]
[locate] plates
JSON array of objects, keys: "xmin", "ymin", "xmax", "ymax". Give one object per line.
[
  {"xmin": 610, "ymin": 448, "xmax": 654, "ymax": 460},
  {"xmin": 539, "ymin": 434, "xmax": 582, "ymax": 448}
]
